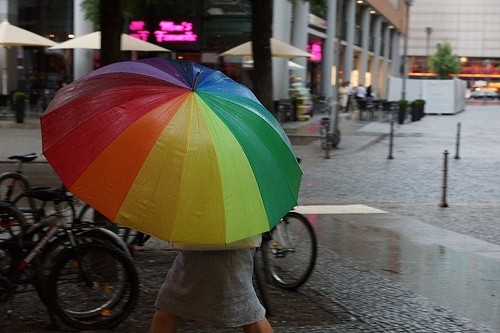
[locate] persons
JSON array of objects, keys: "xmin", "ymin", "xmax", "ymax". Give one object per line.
[
  {"xmin": 28, "ymin": 71, "xmax": 69, "ymax": 118},
  {"xmin": 148, "ymin": 234, "xmax": 275, "ymax": 333},
  {"xmin": 338, "ymin": 81, "xmax": 378, "ymax": 113}
]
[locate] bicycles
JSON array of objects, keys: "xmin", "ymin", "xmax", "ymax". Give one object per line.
[
  {"xmin": 0, "ymin": 151, "xmax": 153, "ymax": 333},
  {"xmin": 260, "ymin": 156, "xmax": 319, "ymax": 290},
  {"xmin": 318, "ymin": 97, "xmax": 340, "ymax": 158}
]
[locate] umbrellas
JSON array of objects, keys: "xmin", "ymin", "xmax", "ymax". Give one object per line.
[
  {"xmin": 1, "ymin": 21, "xmax": 168, "ymax": 50},
  {"xmin": 40, "ymin": 61, "xmax": 304, "ymax": 246},
  {"xmin": 217, "ymin": 38, "xmax": 316, "ymax": 71}
]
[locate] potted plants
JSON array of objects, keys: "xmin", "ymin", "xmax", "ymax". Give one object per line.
[
  {"xmin": 399, "ymin": 100, "xmax": 408, "ymax": 124},
  {"xmin": 13, "ymin": 92, "xmax": 27, "ymax": 123},
  {"xmin": 411, "ymin": 99, "xmax": 426, "ymax": 121}
]
